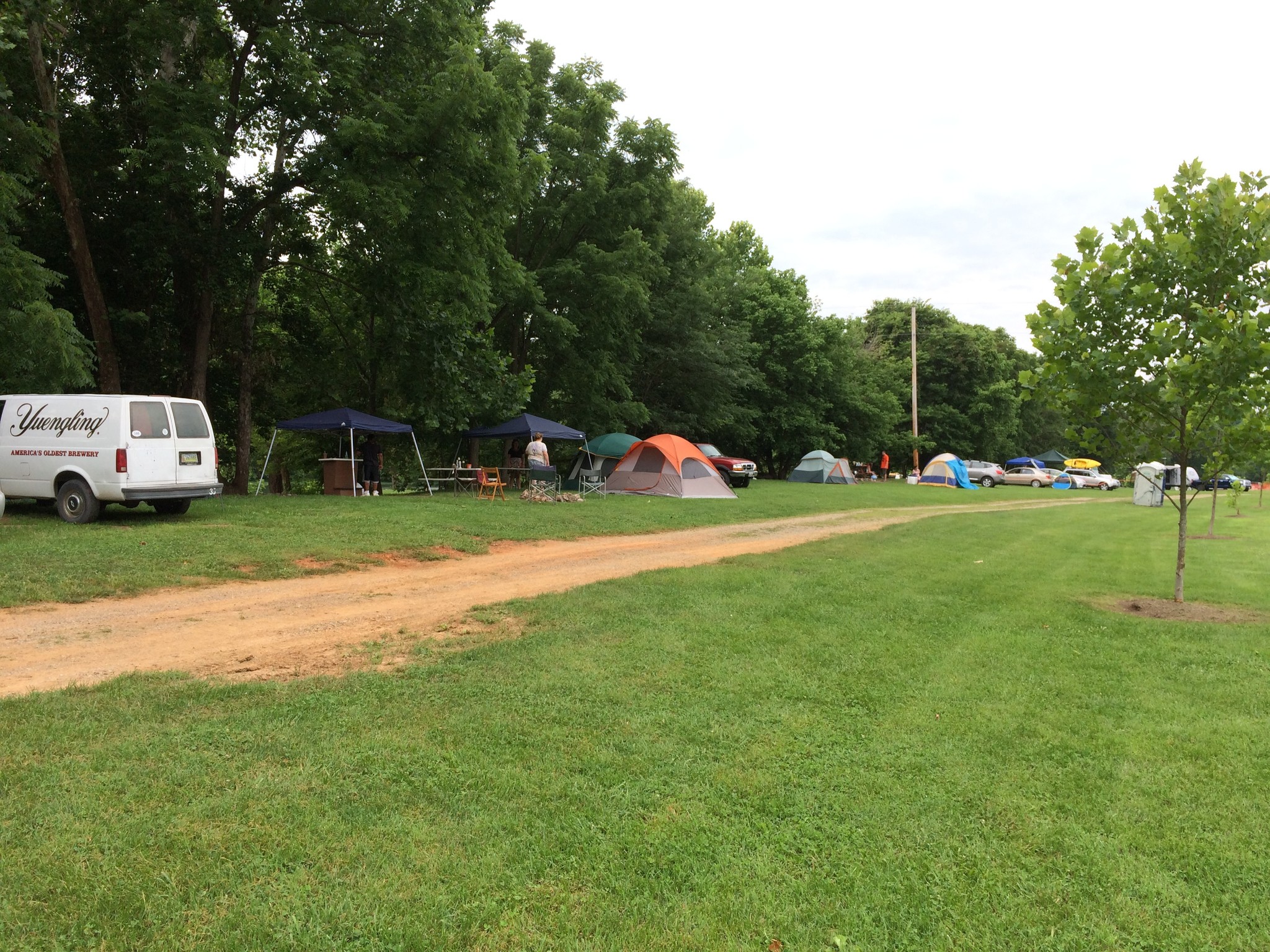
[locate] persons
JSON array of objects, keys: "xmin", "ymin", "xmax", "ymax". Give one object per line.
[
  {"xmin": 911, "ymin": 465, "xmax": 921, "ymax": 484},
  {"xmin": 866, "ymin": 461, "xmax": 880, "ymax": 481},
  {"xmin": 525, "ymin": 432, "xmax": 550, "ymax": 498},
  {"xmin": 508, "ymin": 439, "xmax": 525, "ymax": 492},
  {"xmin": 355, "ymin": 434, "xmax": 384, "ymax": 496},
  {"xmin": 881, "ymin": 450, "xmax": 889, "ymax": 482}
]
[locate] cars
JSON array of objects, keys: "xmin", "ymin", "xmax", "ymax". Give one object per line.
[
  {"xmin": 1189, "ymin": 473, "xmax": 1252, "ymax": 491},
  {"xmin": 1040, "ymin": 469, "xmax": 1087, "ymax": 488},
  {"xmin": 1003, "ymin": 467, "xmax": 1054, "ymax": 488}
]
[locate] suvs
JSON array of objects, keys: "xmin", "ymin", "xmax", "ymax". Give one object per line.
[
  {"xmin": 689, "ymin": 443, "xmax": 759, "ymax": 488},
  {"xmin": 1063, "ymin": 469, "xmax": 1120, "ymax": 490},
  {"xmin": 962, "ymin": 459, "xmax": 1006, "ymax": 487}
]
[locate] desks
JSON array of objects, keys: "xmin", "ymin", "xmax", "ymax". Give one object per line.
[
  {"xmin": 426, "ymin": 468, "xmax": 482, "ymax": 497},
  {"xmin": 497, "ymin": 468, "xmax": 531, "ymax": 491},
  {"xmin": 854, "ymin": 466, "xmax": 867, "ymax": 473}
]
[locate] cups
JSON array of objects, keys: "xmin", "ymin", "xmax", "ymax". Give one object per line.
[{"xmin": 323, "ymin": 453, "xmax": 327, "ymax": 458}]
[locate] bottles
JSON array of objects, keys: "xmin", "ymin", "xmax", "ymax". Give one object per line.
[
  {"xmin": 458, "ymin": 457, "xmax": 460, "ymax": 462},
  {"xmin": 462, "ymin": 461, "xmax": 465, "ymax": 468}
]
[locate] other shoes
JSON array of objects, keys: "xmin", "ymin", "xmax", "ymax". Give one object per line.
[
  {"xmin": 364, "ymin": 491, "xmax": 370, "ymax": 496},
  {"xmin": 372, "ymin": 491, "xmax": 379, "ymax": 496}
]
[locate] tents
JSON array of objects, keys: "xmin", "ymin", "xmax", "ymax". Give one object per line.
[
  {"xmin": 598, "ymin": 433, "xmax": 739, "ymax": 499},
  {"xmin": 919, "ymin": 453, "xmax": 977, "ymax": 489},
  {"xmin": 1032, "ymin": 449, "xmax": 1071, "ymax": 468},
  {"xmin": 255, "ymin": 407, "xmax": 433, "ymax": 498},
  {"xmin": 445, "ymin": 413, "xmax": 599, "ymax": 499},
  {"xmin": 561, "ymin": 432, "xmax": 642, "ymax": 492},
  {"xmin": 1004, "ymin": 455, "xmax": 1046, "ymax": 471},
  {"xmin": 785, "ymin": 450, "xmax": 858, "ymax": 484}
]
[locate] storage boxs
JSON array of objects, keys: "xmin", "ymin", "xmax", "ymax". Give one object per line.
[{"xmin": 907, "ymin": 476, "xmax": 918, "ymax": 485}]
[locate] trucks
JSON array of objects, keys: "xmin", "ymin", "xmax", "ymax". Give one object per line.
[{"xmin": 1162, "ymin": 464, "xmax": 1202, "ymax": 490}]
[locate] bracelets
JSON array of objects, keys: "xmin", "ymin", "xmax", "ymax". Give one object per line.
[{"xmin": 521, "ymin": 463, "xmax": 525, "ymax": 465}]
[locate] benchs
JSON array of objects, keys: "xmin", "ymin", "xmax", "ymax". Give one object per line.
[{"xmin": 418, "ymin": 478, "xmax": 497, "ymax": 498}]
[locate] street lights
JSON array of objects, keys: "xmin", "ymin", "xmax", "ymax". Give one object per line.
[{"xmin": 1163, "ymin": 458, "xmax": 1167, "ymax": 465}]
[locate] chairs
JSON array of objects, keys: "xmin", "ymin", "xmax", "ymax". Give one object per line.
[
  {"xmin": 477, "ymin": 466, "xmax": 507, "ymax": 502},
  {"xmin": 579, "ymin": 468, "xmax": 606, "ymax": 501},
  {"xmin": 527, "ymin": 464, "xmax": 561, "ymax": 506}
]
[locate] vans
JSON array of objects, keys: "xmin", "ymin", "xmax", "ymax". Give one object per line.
[{"xmin": 0, "ymin": 393, "xmax": 223, "ymax": 523}]
